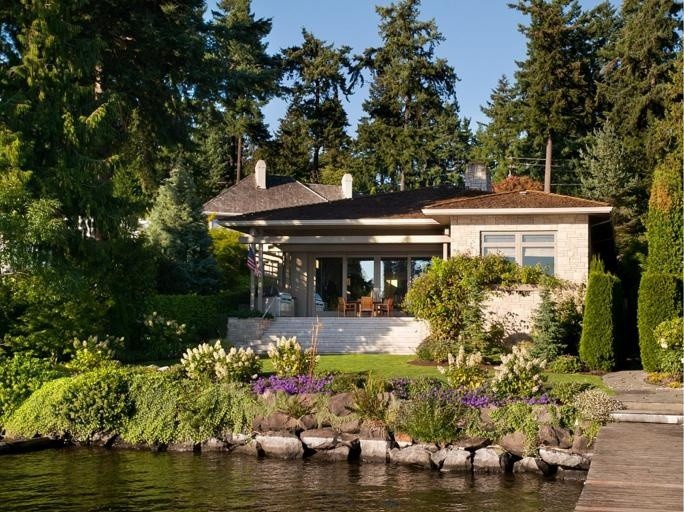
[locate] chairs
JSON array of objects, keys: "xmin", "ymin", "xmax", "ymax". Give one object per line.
[{"xmin": 337, "ymin": 295, "xmax": 393, "ymax": 317}]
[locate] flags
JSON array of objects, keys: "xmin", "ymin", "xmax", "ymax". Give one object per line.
[{"xmin": 246, "ymin": 246, "xmax": 260, "ymax": 278}]
